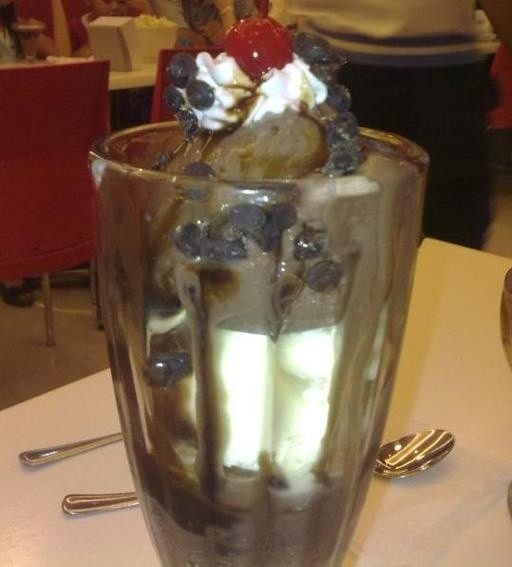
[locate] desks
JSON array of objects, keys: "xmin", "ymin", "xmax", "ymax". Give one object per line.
[
  {"xmin": 0, "ymin": 235, "xmax": 512, "ymax": 566},
  {"xmin": 106, "ymin": 67, "xmax": 156, "ymax": 90}
]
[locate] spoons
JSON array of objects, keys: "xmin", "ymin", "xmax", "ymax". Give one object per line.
[{"xmin": 62, "ymin": 428, "xmax": 456, "ymax": 517}]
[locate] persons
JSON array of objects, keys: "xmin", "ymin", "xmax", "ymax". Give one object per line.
[{"xmin": 266, "ymin": 1, "xmax": 512, "ymax": 251}]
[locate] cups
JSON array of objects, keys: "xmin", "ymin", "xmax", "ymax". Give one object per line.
[
  {"xmin": 84, "ymin": 113, "xmax": 430, "ymax": 567},
  {"xmin": 10, "ymin": 23, "xmax": 46, "ymax": 65}
]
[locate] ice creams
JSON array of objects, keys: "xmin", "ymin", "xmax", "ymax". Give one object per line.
[{"xmin": 101, "ymin": 13, "xmax": 425, "ymax": 505}]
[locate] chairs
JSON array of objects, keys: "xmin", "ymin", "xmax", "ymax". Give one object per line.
[
  {"xmin": 1, "ymin": 60, "xmax": 112, "ymax": 345},
  {"xmin": 150, "ymin": 46, "xmax": 222, "ymax": 123}
]
